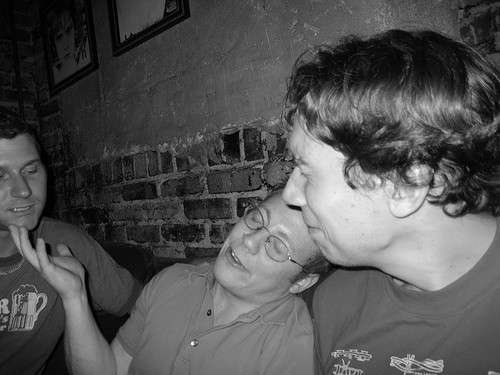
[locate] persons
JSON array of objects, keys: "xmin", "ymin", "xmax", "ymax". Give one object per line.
[
  {"xmin": 0, "ymin": 104, "xmax": 145, "ymax": 375},
  {"xmin": 9, "ymin": 177, "xmax": 333, "ymax": 375},
  {"xmin": 281, "ymin": 28, "xmax": 500, "ymax": 375}
]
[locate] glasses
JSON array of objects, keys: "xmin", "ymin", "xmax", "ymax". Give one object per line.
[{"xmin": 243, "ymin": 202, "xmax": 319, "ymax": 277}]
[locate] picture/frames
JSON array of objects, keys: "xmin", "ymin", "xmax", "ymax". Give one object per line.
[
  {"xmin": 106, "ymin": 0, "xmax": 190, "ymax": 57},
  {"xmin": 44, "ymin": 0, "xmax": 100, "ymax": 97}
]
